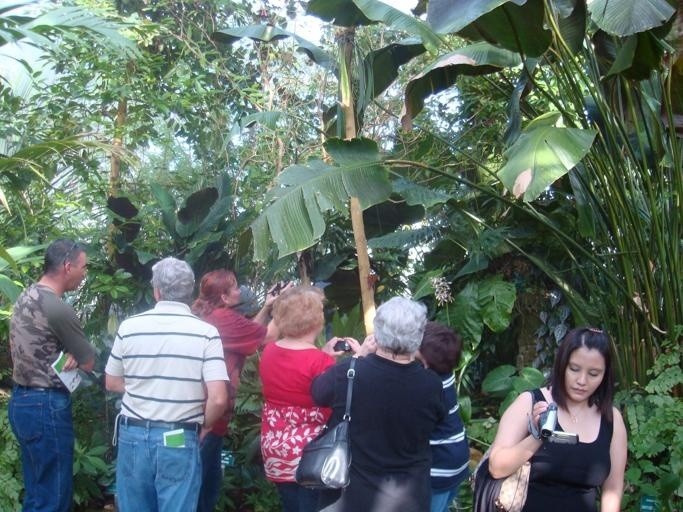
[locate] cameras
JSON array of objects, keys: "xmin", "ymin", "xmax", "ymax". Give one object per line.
[
  {"xmin": 538, "ymin": 401, "xmax": 579, "ymax": 447},
  {"xmin": 333, "ymin": 341, "xmax": 351, "ymax": 352},
  {"xmin": 271, "ymin": 278, "xmax": 290, "ymax": 297}
]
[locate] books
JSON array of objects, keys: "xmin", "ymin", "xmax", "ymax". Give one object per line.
[
  {"xmin": 162, "ymin": 428, "xmax": 186, "ymax": 448},
  {"xmin": 51, "ymin": 350, "xmax": 82, "ymax": 392}
]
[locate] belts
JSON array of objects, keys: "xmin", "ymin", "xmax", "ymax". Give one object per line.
[
  {"xmin": 119, "ymin": 415, "xmax": 197, "ymax": 433},
  {"xmin": 21, "ymin": 385, "xmax": 63, "ymax": 393}
]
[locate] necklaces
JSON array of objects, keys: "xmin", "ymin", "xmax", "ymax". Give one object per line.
[
  {"xmin": 284, "ymin": 336, "xmax": 316, "ymax": 347},
  {"xmin": 562, "ymin": 400, "xmax": 586, "ymax": 425}
]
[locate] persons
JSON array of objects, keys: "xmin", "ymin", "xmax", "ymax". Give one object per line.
[
  {"xmin": 104, "ymin": 255, "xmax": 231, "ymax": 512},
  {"xmin": 9, "ymin": 237, "xmax": 96, "ymax": 512},
  {"xmin": 421, "ymin": 324, "xmax": 470, "ymax": 512},
  {"xmin": 260, "ymin": 286, "xmax": 360, "ymax": 512},
  {"xmin": 192, "ymin": 268, "xmax": 294, "ymax": 512},
  {"xmin": 313, "ymin": 296, "xmax": 448, "ymax": 512},
  {"xmin": 490, "ymin": 330, "xmax": 626, "ymax": 512}
]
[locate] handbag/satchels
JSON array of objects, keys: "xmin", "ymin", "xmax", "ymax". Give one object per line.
[
  {"xmin": 470, "ymin": 388, "xmax": 531, "ymax": 512},
  {"xmin": 295, "ymin": 357, "xmax": 358, "ymax": 489}
]
